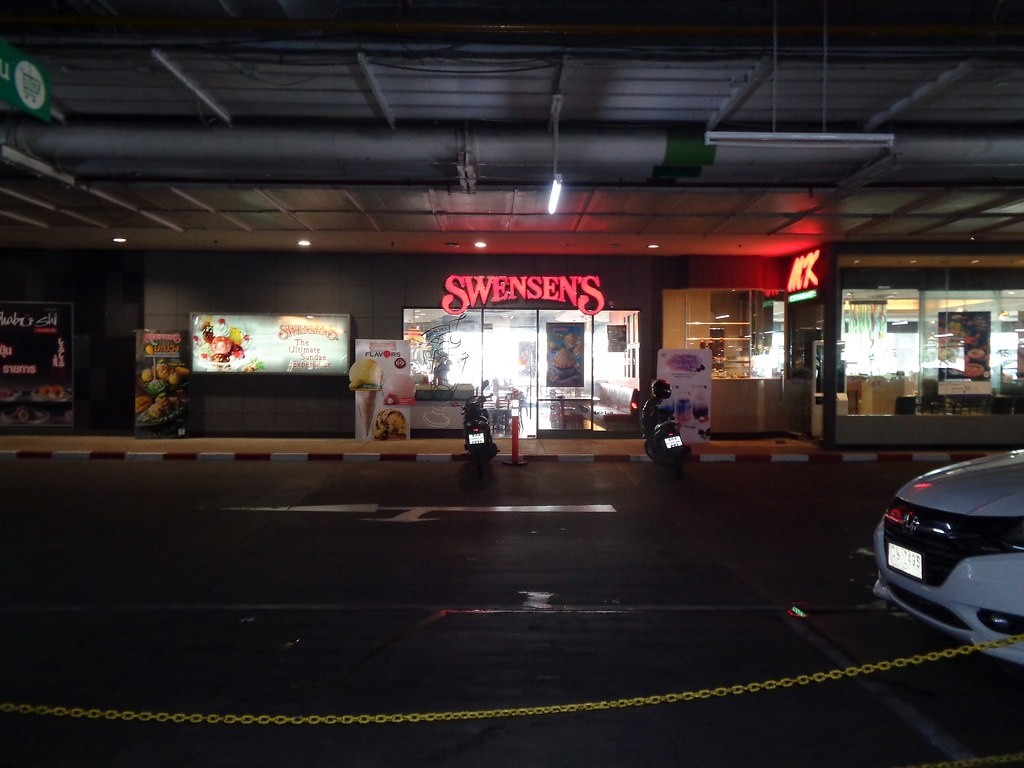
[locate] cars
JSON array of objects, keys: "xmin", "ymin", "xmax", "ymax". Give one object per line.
[{"xmin": 873, "ymin": 445, "xmax": 1024, "ymax": 671}]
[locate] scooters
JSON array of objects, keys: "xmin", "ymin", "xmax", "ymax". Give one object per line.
[{"xmin": 642, "ymin": 378, "xmax": 692, "ymax": 473}]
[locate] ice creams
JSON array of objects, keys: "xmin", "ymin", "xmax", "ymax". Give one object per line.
[
  {"xmin": 194, "ymin": 318, "xmax": 253, "ymax": 363},
  {"xmin": 349, "ymin": 358, "xmax": 416, "ymax": 440}
]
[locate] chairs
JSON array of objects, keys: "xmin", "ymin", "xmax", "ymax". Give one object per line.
[{"xmin": 559, "ymin": 379, "xmax": 1024, "ymax": 431}]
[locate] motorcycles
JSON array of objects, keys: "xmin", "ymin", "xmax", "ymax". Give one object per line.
[{"xmin": 451, "ymin": 379, "xmax": 501, "ymax": 485}]
